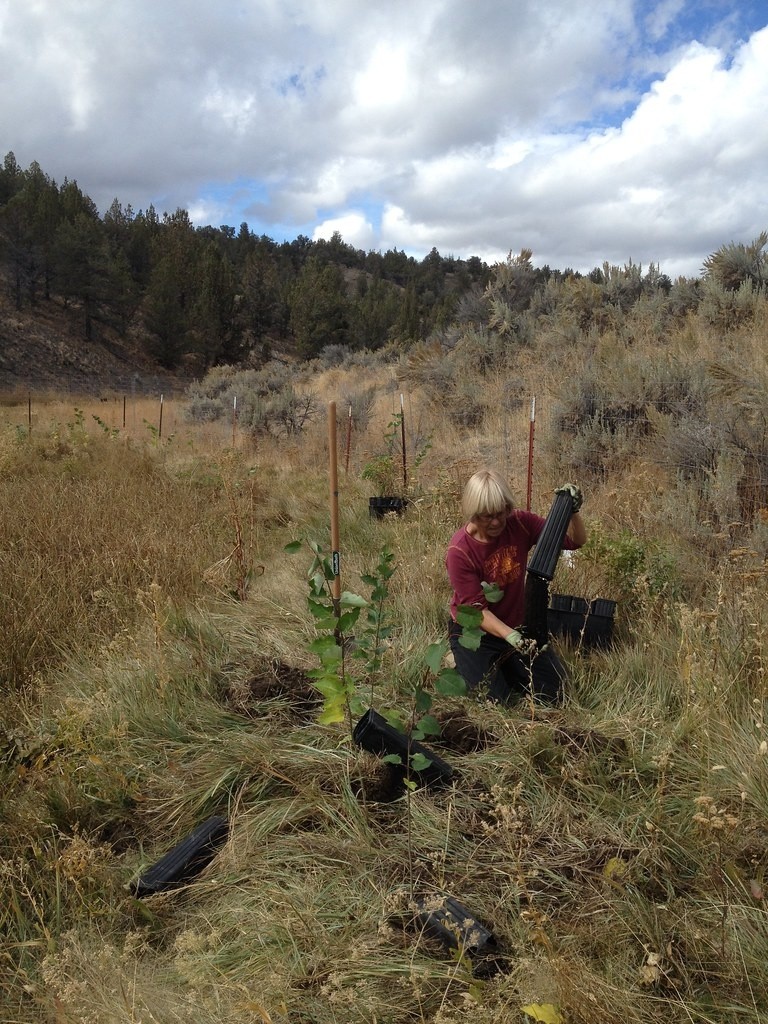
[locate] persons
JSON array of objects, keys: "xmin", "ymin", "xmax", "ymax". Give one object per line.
[{"xmin": 444, "ymin": 470, "xmax": 589, "ymax": 710}]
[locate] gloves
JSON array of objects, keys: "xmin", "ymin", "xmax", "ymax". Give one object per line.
[
  {"xmin": 554, "ymin": 484, "xmax": 583, "ymax": 512},
  {"xmin": 506, "ymin": 631, "xmax": 548, "ymax": 657}
]
